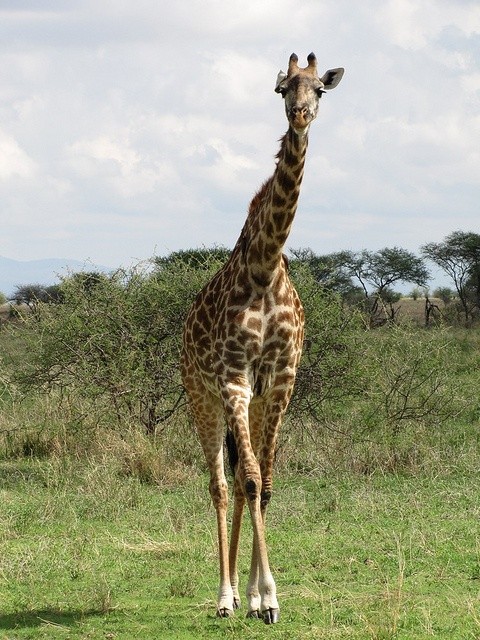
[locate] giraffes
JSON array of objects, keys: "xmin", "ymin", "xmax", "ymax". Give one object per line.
[{"xmin": 180, "ymin": 52, "xmax": 344, "ymax": 624}]
